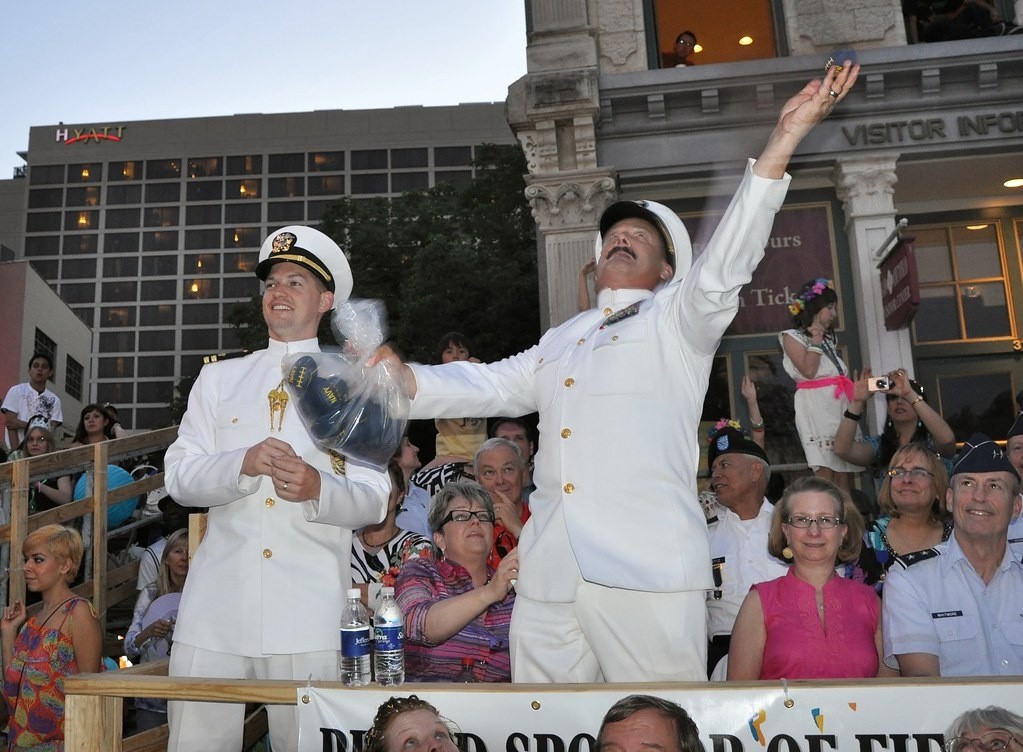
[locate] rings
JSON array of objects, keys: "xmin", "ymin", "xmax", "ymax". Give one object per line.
[
  {"xmin": 897, "ymin": 372, "xmax": 902, "ymax": 375},
  {"xmin": 161, "ymin": 630, "xmax": 163, "ymax": 634},
  {"xmin": 496, "ymin": 507, "xmax": 500, "ymax": 512},
  {"xmin": 829, "ymin": 90, "xmax": 838, "ymax": 98},
  {"xmin": 282, "ymin": 481, "xmax": 288, "ymax": 491}
]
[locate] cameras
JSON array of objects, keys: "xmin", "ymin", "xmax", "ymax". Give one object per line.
[{"xmin": 867, "ymin": 376, "xmax": 889, "ymax": 391}]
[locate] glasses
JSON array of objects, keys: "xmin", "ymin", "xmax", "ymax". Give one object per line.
[
  {"xmin": 785, "ymin": 514, "xmax": 845, "ymax": 529},
  {"xmin": 435, "ymin": 509, "xmax": 495, "ymax": 531},
  {"xmin": 886, "ymin": 466, "xmax": 937, "ymax": 479},
  {"xmin": 944, "ymin": 731, "xmax": 1023, "ymax": 752},
  {"xmin": 495, "ymin": 530, "xmax": 513, "ymax": 559},
  {"xmin": 677, "ymin": 39, "xmax": 695, "ymax": 46},
  {"xmin": 27, "ymin": 435, "xmax": 49, "ymax": 441}
]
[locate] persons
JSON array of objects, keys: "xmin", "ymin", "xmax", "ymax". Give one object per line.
[
  {"xmin": 0, "ymin": 367, "xmax": 1023, "ymax": 752},
  {"xmin": 912, "ymin": 0, "xmax": 1023, "ymax": 42},
  {"xmin": 662, "ymin": 30, "xmax": 697, "ymax": 68},
  {"xmin": 941, "ymin": 704, "xmax": 1023, "ymax": 752},
  {"xmin": 590, "ymin": 694, "xmax": 705, "ymax": 752},
  {"xmin": 775, "ymin": 278, "xmax": 866, "ymax": 502},
  {"xmin": 364, "ymin": 694, "xmax": 460, "ymax": 752},
  {"xmin": 0, "ymin": 353, "xmax": 63, "ymax": 453},
  {"xmin": 160, "ymin": 223, "xmax": 393, "ymax": 752},
  {"xmin": 348, "ymin": 61, "xmax": 864, "ymax": 681},
  {"xmin": 434, "ymin": 333, "xmax": 488, "ymax": 455}
]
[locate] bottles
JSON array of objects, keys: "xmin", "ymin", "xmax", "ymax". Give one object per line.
[
  {"xmin": 453, "ymin": 658, "xmax": 479, "ymax": 684},
  {"xmin": 373, "ymin": 588, "xmax": 406, "ymax": 687},
  {"xmin": 339, "ymin": 588, "xmax": 372, "ymax": 688}
]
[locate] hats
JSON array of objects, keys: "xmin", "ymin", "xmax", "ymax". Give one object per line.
[
  {"xmin": 886, "ymin": 378, "xmax": 927, "ymax": 402},
  {"xmin": 1006, "ymin": 412, "xmax": 1023, "ymax": 439},
  {"xmin": 947, "ymin": 430, "xmax": 1020, "ymax": 482},
  {"xmin": 103, "ymin": 401, "xmax": 119, "ymax": 413},
  {"xmin": 708, "ymin": 425, "xmax": 770, "ymax": 472},
  {"xmin": 254, "ymin": 225, "xmax": 354, "ymax": 311},
  {"xmin": 596, "ymin": 199, "xmax": 693, "ymax": 293}
]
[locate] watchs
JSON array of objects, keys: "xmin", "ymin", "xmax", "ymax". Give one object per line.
[{"xmin": 911, "ymin": 395, "xmax": 923, "ymax": 407}]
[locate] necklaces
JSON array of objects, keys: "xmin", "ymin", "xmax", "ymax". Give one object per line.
[
  {"xmin": 818, "ymin": 601, "xmax": 824, "ymax": 610},
  {"xmin": 362, "ymin": 528, "xmax": 401, "ymax": 548}
]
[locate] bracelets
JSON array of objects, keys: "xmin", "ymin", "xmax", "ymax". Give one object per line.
[
  {"xmin": 843, "ymin": 409, "xmax": 862, "ymax": 422},
  {"xmin": 806, "ymin": 342, "xmax": 824, "ymax": 355},
  {"xmin": 38, "ymin": 481, "xmax": 41, "ymax": 493},
  {"xmin": 749, "ymin": 416, "xmax": 765, "ymax": 433}
]
